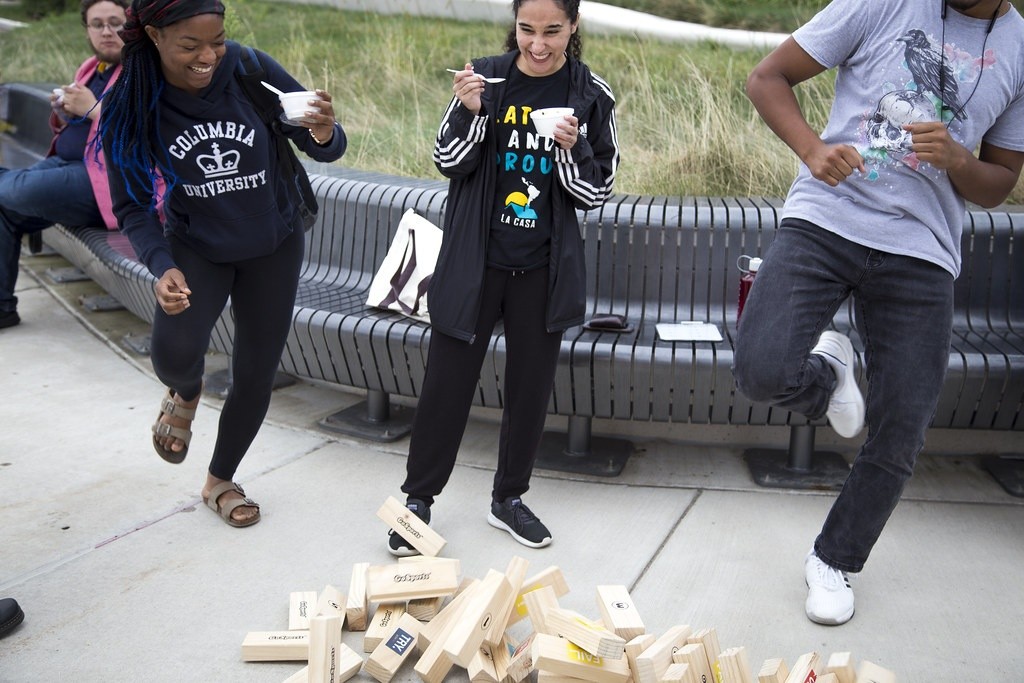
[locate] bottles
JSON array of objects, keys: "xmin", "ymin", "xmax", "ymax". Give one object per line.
[{"xmin": 736, "ymin": 255, "xmax": 763, "ymax": 332}]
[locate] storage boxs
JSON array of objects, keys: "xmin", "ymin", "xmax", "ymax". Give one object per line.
[{"xmin": 241, "ymin": 494, "xmax": 899, "ymax": 683}]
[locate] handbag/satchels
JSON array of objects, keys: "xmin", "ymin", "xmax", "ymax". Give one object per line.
[{"xmin": 365, "ymin": 207, "xmax": 443, "ymax": 324}]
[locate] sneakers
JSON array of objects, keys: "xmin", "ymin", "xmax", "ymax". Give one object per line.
[
  {"xmin": 387, "ymin": 500, "xmax": 431, "ymax": 556},
  {"xmin": 811, "ymin": 330, "xmax": 864, "ymax": 438},
  {"xmin": 486, "ymin": 496, "xmax": 553, "ymax": 549},
  {"xmin": 805, "ymin": 545, "xmax": 855, "ymax": 627}
]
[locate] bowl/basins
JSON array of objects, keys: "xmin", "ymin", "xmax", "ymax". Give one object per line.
[
  {"xmin": 53, "ymin": 89, "xmax": 66, "ymax": 101},
  {"xmin": 530, "ymin": 108, "xmax": 574, "ymax": 137},
  {"xmin": 278, "ymin": 91, "xmax": 323, "ymax": 121}
]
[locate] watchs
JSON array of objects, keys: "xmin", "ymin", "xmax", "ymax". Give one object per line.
[{"xmin": 309, "ymin": 129, "xmax": 333, "ymax": 145}]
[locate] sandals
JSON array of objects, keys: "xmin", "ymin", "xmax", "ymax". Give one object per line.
[
  {"xmin": 201, "ymin": 477, "xmax": 261, "ymax": 528},
  {"xmin": 152, "ymin": 387, "xmax": 202, "ymax": 465}
]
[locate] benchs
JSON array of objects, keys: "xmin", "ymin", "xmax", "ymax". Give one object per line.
[{"xmin": 0, "ymin": 83, "xmax": 1024, "ymax": 499}]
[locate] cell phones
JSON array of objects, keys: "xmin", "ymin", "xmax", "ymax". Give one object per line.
[{"xmin": 583, "ymin": 320, "xmax": 635, "ymax": 332}]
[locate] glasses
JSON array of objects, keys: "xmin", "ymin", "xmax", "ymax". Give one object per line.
[{"xmin": 84, "ymin": 20, "xmax": 124, "ymax": 33}]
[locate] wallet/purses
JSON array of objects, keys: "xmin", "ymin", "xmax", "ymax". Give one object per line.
[{"xmin": 588, "ymin": 313, "xmax": 629, "ymax": 328}]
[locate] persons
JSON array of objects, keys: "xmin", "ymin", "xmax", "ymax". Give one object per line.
[
  {"xmin": 0, "ymin": 597, "xmax": 25, "ymax": 638},
  {"xmin": 387, "ymin": 1, "xmax": 620, "ymax": 558},
  {"xmin": 0, "ymin": 0, "xmax": 165, "ymax": 329},
  {"xmin": 100, "ymin": 0, "xmax": 348, "ymax": 527},
  {"xmin": 730, "ymin": 0, "xmax": 1024, "ymax": 628}
]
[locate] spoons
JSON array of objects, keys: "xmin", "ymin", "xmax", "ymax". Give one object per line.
[{"xmin": 446, "ymin": 69, "xmax": 506, "ymax": 84}]
